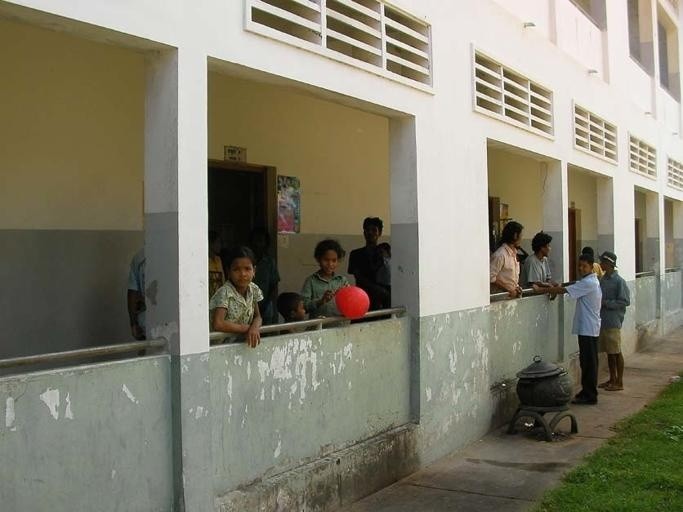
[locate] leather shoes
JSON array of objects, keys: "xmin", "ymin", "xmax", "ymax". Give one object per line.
[
  {"xmin": 575, "ymin": 390, "xmax": 597, "ymax": 398},
  {"xmin": 571, "ymin": 398, "xmax": 597, "ymax": 405}
]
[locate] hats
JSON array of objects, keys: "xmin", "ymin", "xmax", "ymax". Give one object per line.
[{"xmin": 598, "ymin": 251, "xmax": 616, "ymax": 268}]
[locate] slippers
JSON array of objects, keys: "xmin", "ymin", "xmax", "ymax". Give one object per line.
[
  {"xmin": 598, "ymin": 382, "xmax": 609, "ymax": 388},
  {"xmin": 606, "ymin": 384, "xmax": 622, "ymax": 390}
]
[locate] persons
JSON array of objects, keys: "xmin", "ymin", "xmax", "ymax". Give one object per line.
[
  {"xmin": 532, "ymin": 254, "xmax": 602, "ymax": 405},
  {"xmin": 598, "ymin": 251, "xmax": 630, "ymax": 391},
  {"xmin": 523, "ymin": 230, "xmax": 561, "ymax": 300},
  {"xmin": 127, "ymin": 217, "xmax": 391, "ymax": 357},
  {"xmin": 490, "ymin": 221, "xmax": 524, "ymax": 302},
  {"xmin": 581, "ymin": 246, "xmax": 603, "ymax": 278}
]
